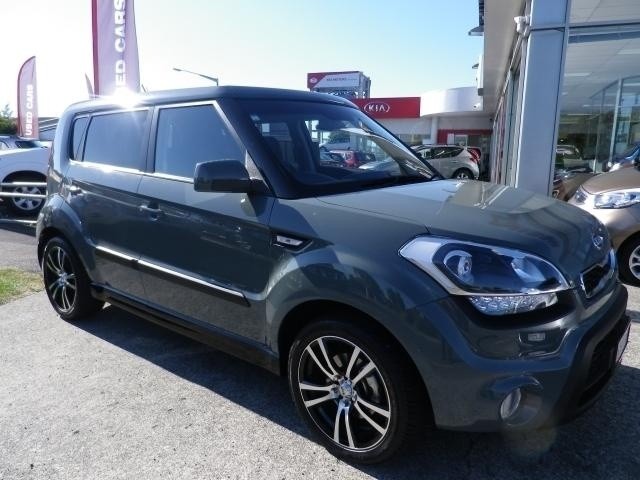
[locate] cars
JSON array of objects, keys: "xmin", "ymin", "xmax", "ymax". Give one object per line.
[
  {"xmin": 553, "ymin": 144, "xmax": 640, "ymax": 203},
  {"xmin": 0, "ymin": 118, "xmax": 60, "ymax": 218},
  {"xmin": 319, "ymin": 149, "xmax": 377, "ymax": 169},
  {"xmin": 567, "ymin": 163, "xmax": 640, "ymax": 288}
]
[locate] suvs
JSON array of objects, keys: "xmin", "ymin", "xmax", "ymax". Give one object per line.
[
  {"xmin": 34, "ymin": 81, "xmax": 635, "ymax": 469},
  {"xmin": 358, "ymin": 144, "xmax": 481, "ymax": 181}
]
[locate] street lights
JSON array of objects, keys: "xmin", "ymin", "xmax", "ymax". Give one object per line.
[{"xmin": 171, "ymin": 66, "xmax": 220, "ymax": 86}]
